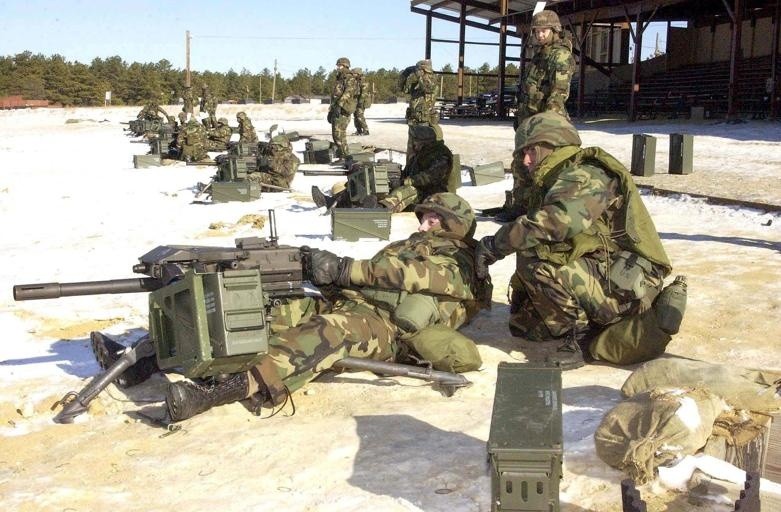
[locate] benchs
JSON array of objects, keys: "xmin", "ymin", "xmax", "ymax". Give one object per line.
[{"xmin": 436, "ymin": 51, "xmax": 781, "ymax": 119}]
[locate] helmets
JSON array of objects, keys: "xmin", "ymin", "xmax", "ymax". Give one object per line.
[
  {"xmin": 236, "ymin": 112, "xmax": 246, "ymax": 120},
  {"xmin": 335, "ymin": 58, "xmax": 351, "ymax": 68},
  {"xmin": 408, "ymin": 125, "xmax": 437, "ymax": 144},
  {"xmin": 415, "ymin": 60, "xmax": 434, "ymax": 75},
  {"xmin": 350, "ymin": 66, "xmax": 363, "ymax": 75},
  {"xmin": 201, "ymin": 82, "xmax": 209, "ymax": 89},
  {"xmin": 530, "ymin": 9, "xmax": 563, "ymax": 36},
  {"xmin": 416, "ymin": 193, "xmax": 477, "ymax": 241},
  {"xmin": 268, "ymin": 135, "xmax": 290, "ymax": 150},
  {"xmin": 178, "ymin": 112, "xmax": 186, "ymax": 118},
  {"xmin": 217, "ymin": 118, "xmax": 228, "ymax": 127},
  {"xmin": 513, "ymin": 112, "xmax": 582, "ymax": 160}
]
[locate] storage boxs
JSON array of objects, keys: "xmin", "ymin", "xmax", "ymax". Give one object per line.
[
  {"xmin": 468, "ymin": 159, "xmax": 509, "ymax": 187},
  {"xmin": 440, "ymin": 150, "xmax": 464, "ymax": 192},
  {"xmin": 481, "ymin": 358, "xmax": 568, "ymax": 512},
  {"xmin": 144, "ymin": 267, "xmax": 273, "ymax": 384},
  {"xmin": 325, "ymin": 204, "xmax": 395, "ymax": 245},
  {"xmin": 627, "ymin": 130, "xmax": 659, "ymax": 179},
  {"xmin": 131, "ymin": 120, "xmax": 335, "ymax": 200},
  {"xmin": 667, "ymin": 129, "xmax": 695, "ymax": 177},
  {"xmin": 342, "ymin": 166, "xmax": 394, "ymax": 204},
  {"xmin": 342, "ymin": 150, "xmax": 378, "ymax": 168}
]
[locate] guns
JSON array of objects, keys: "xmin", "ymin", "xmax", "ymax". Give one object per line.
[
  {"xmin": 13, "ymin": 210, "xmax": 321, "ymax": 420},
  {"xmin": 129, "ymin": 132, "xmax": 183, "ymax": 160},
  {"xmin": 186, "ymin": 142, "xmax": 259, "ymax": 183},
  {"xmin": 302, "ymin": 152, "xmax": 401, "ymax": 209},
  {"xmin": 121, "ymin": 121, "xmax": 162, "ymax": 136}
]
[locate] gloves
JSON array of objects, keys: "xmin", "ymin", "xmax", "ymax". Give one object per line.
[
  {"xmin": 332, "ymin": 105, "xmax": 342, "ymax": 118},
  {"xmin": 327, "ymin": 110, "xmax": 333, "ymax": 123},
  {"xmin": 257, "ymin": 153, "xmax": 270, "ymax": 167},
  {"xmin": 403, "ymin": 66, "xmax": 417, "ymax": 77},
  {"xmin": 472, "ymin": 236, "xmax": 504, "ymax": 279},
  {"xmin": 301, "ymin": 244, "xmax": 355, "ymax": 290}
]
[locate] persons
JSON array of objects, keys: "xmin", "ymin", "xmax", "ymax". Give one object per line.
[
  {"xmin": 494, "ymin": 9, "xmax": 578, "ymax": 223},
  {"xmin": 203, "ymin": 92, "xmax": 219, "ymax": 117},
  {"xmin": 181, "ymin": 89, "xmax": 195, "ymax": 116},
  {"xmin": 135, "ymin": 100, "xmax": 233, "ymax": 165},
  {"xmin": 244, "ymin": 135, "xmax": 302, "ymax": 191},
  {"xmin": 327, "ymin": 57, "xmax": 361, "ymax": 166},
  {"xmin": 226, "ymin": 111, "xmax": 260, "ymax": 150},
  {"xmin": 88, "ymin": 190, "xmax": 496, "ymax": 424},
  {"xmin": 352, "ymin": 73, "xmax": 371, "ymax": 136},
  {"xmin": 471, "ymin": 107, "xmax": 675, "ymax": 372},
  {"xmin": 403, "ymin": 59, "xmax": 442, "ymax": 165},
  {"xmin": 311, "ymin": 124, "xmax": 455, "ymax": 212}
]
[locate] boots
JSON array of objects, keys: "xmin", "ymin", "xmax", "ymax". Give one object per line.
[
  {"xmin": 164, "ymin": 370, "xmax": 250, "ymax": 422},
  {"xmin": 352, "ymin": 127, "xmax": 369, "ymax": 136},
  {"xmin": 495, "ymin": 203, "xmax": 528, "ymax": 222},
  {"xmin": 360, "ymin": 194, "xmax": 387, "ymax": 210},
  {"xmin": 312, "ymin": 186, "xmax": 351, "ymax": 210},
  {"xmin": 331, "ymin": 154, "xmax": 345, "ymax": 165},
  {"xmin": 91, "ymin": 331, "xmax": 158, "ymax": 387},
  {"xmin": 543, "ymin": 324, "xmax": 599, "ymax": 370}
]
